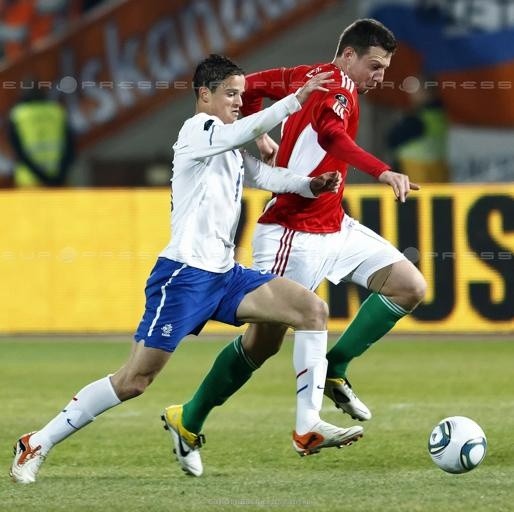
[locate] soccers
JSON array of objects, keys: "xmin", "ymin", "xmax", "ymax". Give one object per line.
[{"xmin": 428, "ymin": 416, "xmax": 486, "ymax": 474}]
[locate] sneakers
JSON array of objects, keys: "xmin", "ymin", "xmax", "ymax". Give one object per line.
[
  {"xmin": 324, "ymin": 376, "xmax": 372, "ymax": 421},
  {"xmin": 292, "ymin": 420, "xmax": 364, "ymax": 457},
  {"xmin": 160, "ymin": 404, "xmax": 207, "ymax": 477},
  {"xmin": 10, "ymin": 430, "xmax": 47, "ymax": 484}
]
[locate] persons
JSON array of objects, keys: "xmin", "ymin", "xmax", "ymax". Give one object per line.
[
  {"xmin": 384, "ymin": 75, "xmax": 450, "ymax": 184},
  {"xmin": 8, "ymin": 54, "xmax": 364, "ymax": 485},
  {"xmin": 4, "ymin": 77, "xmax": 77, "ymax": 189},
  {"xmin": 160, "ymin": 17, "xmax": 428, "ymax": 478}
]
